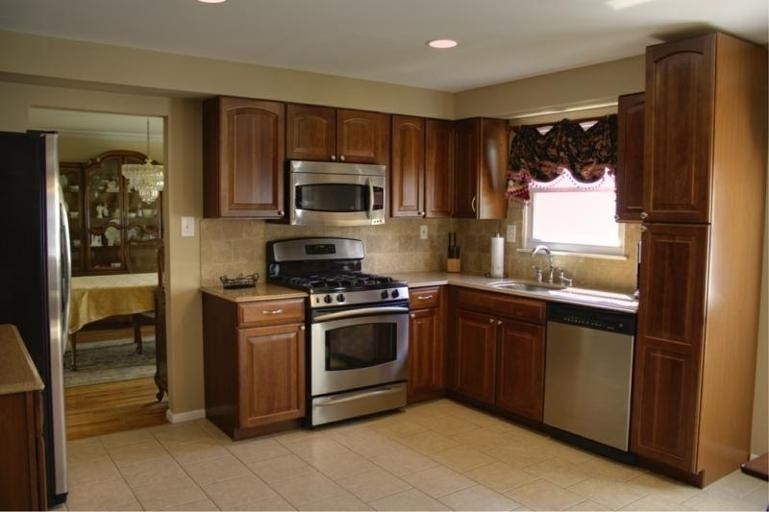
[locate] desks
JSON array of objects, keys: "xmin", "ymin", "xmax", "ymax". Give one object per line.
[{"xmin": 69, "ymin": 272, "xmax": 158, "ymax": 370}]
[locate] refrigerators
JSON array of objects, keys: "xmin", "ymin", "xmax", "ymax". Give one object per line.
[{"xmin": 1, "ymin": 130, "xmax": 70, "ymax": 506}]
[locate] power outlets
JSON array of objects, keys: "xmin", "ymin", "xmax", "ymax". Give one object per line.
[{"xmin": 420, "ymin": 225, "xmax": 427, "ymax": 239}]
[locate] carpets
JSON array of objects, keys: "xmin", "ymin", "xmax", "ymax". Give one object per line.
[{"xmin": 64, "ymin": 335, "xmax": 157, "ymax": 389}]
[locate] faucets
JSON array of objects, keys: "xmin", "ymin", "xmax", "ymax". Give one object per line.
[{"xmin": 530, "ymin": 244, "xmax": 554, "ymax": 283}]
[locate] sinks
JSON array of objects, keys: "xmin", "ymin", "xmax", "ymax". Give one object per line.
[{"xmin": 490, "ymin": 281, "xmax": 560, "ymax": 293}]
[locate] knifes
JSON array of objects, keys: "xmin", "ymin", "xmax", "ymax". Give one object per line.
[{"xmin": 448, "ymin": 231, "xmax": 457, "ymax": 257}]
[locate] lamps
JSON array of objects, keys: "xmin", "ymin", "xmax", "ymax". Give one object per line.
[{"xmin": 122, "ymin": 117, "xmax": 165, "ymax": 206}]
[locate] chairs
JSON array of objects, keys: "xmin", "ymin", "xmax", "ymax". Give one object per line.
[{"xmin": 123, "ymin": 243, "xmax": 159, "ymax": 352}]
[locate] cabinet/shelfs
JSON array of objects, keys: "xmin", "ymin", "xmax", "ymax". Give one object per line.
[
  {"xmin": 0, "ymin": 322, "xmax": 49, "ymax": 511},
  {"xmin": 628, "ymin": 224, "xmax": 763, "ymax": 491},
  {"xmin": 640, "ymin": 32, "xmax": 768, "ymax": 223},
  {"xmin": 391, "ymin": 113, "xmax": 456, "ymax": 217},
  {"xmin": 59, "ymin": 150, "xmax": 162, "ymax": 275},
  {"xmin": 201, "ymin": 292, "xmax": 307, "ymax": 442},
  {"xmin": 616, "ymin": 91, "xmax": 646, "ymax": 223},
  {"xmin": 203, "ymin": 96, "xmax": 286, "ymax": 220},
  {"xmin": 447, "ymin": 283, "xmax": 546, "ymax": 429},
  {"xmin": 408, "ymin": 286, "xmax": 447, "ymax": 406},
  {"xmin": 456, "ymin": 116, "xmax": 510, "ymax": 220},
  {"xmin": 287, "ymin": 102, "xmax": 391, "ymax": 165}
]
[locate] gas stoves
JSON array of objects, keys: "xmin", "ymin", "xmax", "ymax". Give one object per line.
[{"xmin": 277, "ymin": 268, "xmax": 401, "ymax": 294}]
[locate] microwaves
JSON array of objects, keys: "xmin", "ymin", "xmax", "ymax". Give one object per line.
[{"xmin": 284, "ymin": 161, "xmax": 388, "ymax": 226}]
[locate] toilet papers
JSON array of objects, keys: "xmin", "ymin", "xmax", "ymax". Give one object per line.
[{"xmin": 491, "ymin": 238, "xmax": 503, "ymax": 277}]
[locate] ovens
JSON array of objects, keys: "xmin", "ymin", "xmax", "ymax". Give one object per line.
[{"xmin": 309, "ymin": 286, "xmax": 410, "ymax": 428}]
